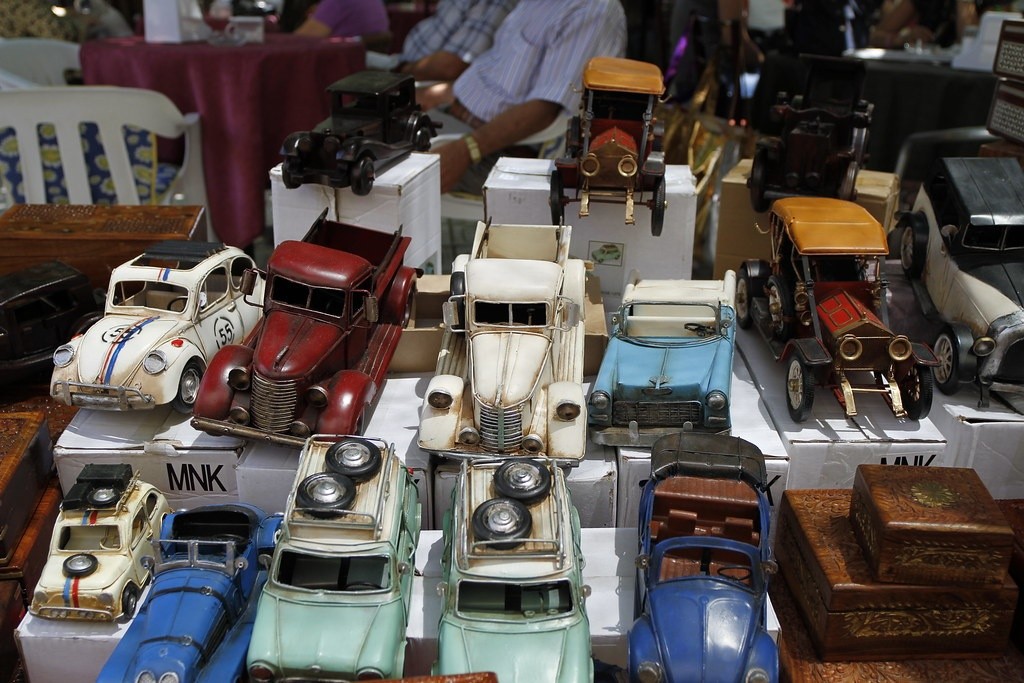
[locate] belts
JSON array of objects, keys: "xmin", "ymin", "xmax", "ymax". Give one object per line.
[{"xmin": 448, "ymin": 100, "xmax": 486, "ymax": 130}]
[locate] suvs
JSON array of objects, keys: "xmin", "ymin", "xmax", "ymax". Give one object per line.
[
  {"xmin": 899, "ymin": 152, "xmax": 1024, "ymax": 396},
  {"xmin": 1, "ymin": 257, "xmax": 105, "ymax": 401},
  {"xmin": 246, "ymin": 433, "xmax": 423, "ymax": 683},
  {"xmin": 735, "ymin": 198, "xmax": 940, "ymax": 424},
  {"xmin": 30, "ymin": 464, "xmax": 167, "ymax": 622},
  {"xmin": 431, "ymin": 457, "xmax": 595, "ymax": 683}
]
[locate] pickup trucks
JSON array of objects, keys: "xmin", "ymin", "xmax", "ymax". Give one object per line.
[{"xmin": 417, "ymin": 221, "xmax": 590, "ymax": 469}]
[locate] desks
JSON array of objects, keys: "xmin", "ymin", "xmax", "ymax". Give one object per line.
[{"xmin": 798, "ymin": 52, "xmax": 997, "ymax": 79}]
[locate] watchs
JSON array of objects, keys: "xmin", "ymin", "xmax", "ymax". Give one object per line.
[{"xmin": 460, "ymin": 133, "xmax": 485, "ymax": 166}]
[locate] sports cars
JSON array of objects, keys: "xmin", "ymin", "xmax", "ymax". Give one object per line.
[
  {"xmin": 97, "ymin": 504, "xmax": 284, "ymax": 683},
  {"xmin": 589, "ymin": 270, "xmax": 738, "ymax": 444}
]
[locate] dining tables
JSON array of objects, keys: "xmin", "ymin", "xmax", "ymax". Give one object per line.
[{"xmin": 79, "ymin": 29, "xmax": 367, "ymax": 249}]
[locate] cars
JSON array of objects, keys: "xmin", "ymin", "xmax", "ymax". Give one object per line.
[
  {"xmin": 749, "ymin": 93, "xmax": 876, "ymax": 213},
  {"xmin": 627, "ymin": 434, "xmax": 782, "ymax": 682},
  {"xmin": 282, "ymin": 71, "xmax": 445, "ymax": 194},
  {"xmin": 549, "ymin": 57, "xmax": 668, "ymax": 237},
  {"xmin": 51, "ymin": 238, "xmax": 269, "ymax": 416}
]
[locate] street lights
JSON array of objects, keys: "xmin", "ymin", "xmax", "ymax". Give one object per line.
[{"xmin": 189, "ymin": 206, "xmax": 424, "ymax": 453}]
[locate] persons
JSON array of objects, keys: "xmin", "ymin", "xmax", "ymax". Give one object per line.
[
  {"xmin": 365, "ymin": 0, "xmax": 518, "ymax": 87},
  {"xmin": 868, "ymin": 0, "xmax": 960, "ymax": 51},
  {"xmin": 296, "ymin": 2, "xmax": 391, "ymax": 41},
  {"xmin": 305, "ymin": 0, "xmax": 630, "ymax": 222}
]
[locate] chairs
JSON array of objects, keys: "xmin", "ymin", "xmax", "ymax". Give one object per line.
[
  {"xmin": 63, "ymin": 520, "xmax": 141, "ymax": 549},
  {"xmin": 172, "ymin": 504, "xmax": 258, "ymax": 556},
  {"xmin": 146, "ymin": 290, "xmax": 225, "ymax": 311},
  {"xmin": 0, "ymin": 86, "xmax": 221, "ymax": 243},
  {"xmin": 656, "ymin": 509, "xmax": 707, "ymax": 581},
  {"xmin": 291, "ymin": 554, "xmax": 341, "ymax": 589},
  {"xmin": 707, "ymin": 517, "xmax": 760, "ymax": 588},
  {"xmin": 0, "ymin": 36, "xmax": 82, "ymax": 86}
]
